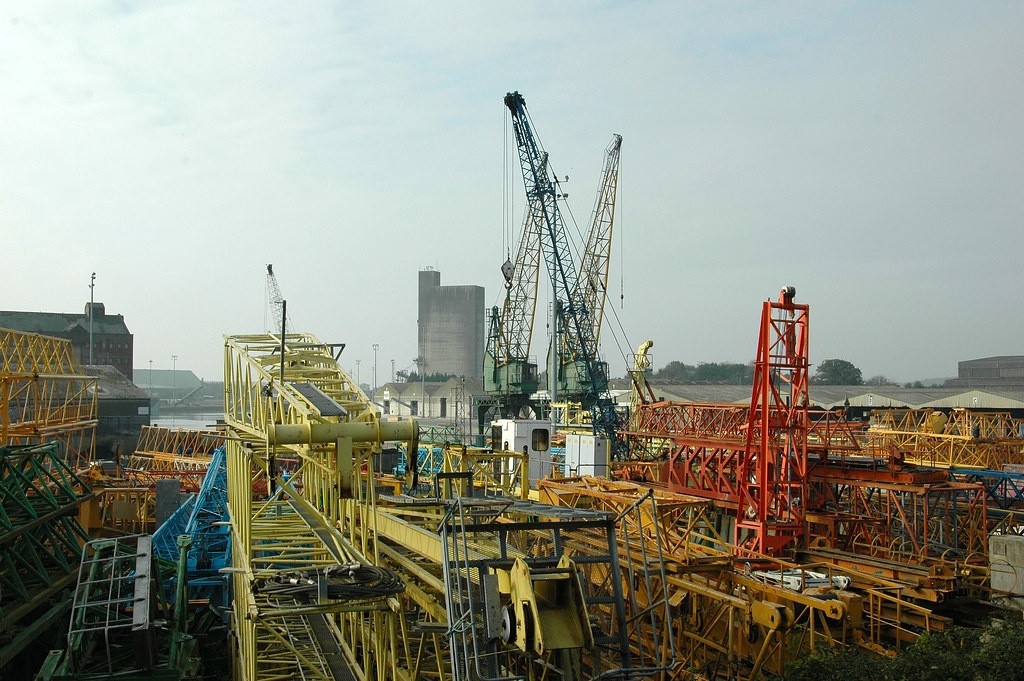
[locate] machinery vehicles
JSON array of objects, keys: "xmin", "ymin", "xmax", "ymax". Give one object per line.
[
  {"xmin": 264, "ymin": 264, "xmax": 298, "ymax": 333},
  {"xmin": 547, "ymin": 133, "xmax": 624, "ymax": 411},
  {"xmin": 482, "ymin": 153, "xmax": 550, "ymax": 419},
  {"xmin": 504, "ymin": 90, "xmax": 627, "ymax": 430}
]
